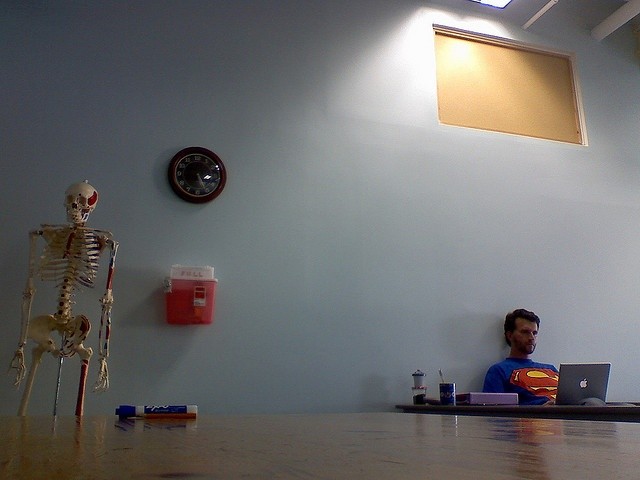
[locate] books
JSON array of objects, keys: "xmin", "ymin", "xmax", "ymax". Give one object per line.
[{"xmin": 456, "ymin": 393, "xmax": 519, "ymax": 405}]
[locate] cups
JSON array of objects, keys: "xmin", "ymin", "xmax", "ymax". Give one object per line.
[
  {"xmin": 412, "ymin": 370, "xmax": 426, "ymax": 387},
  {"xmin": 439, "ymin": 382, "xmax": 455, "ymax": 405}
]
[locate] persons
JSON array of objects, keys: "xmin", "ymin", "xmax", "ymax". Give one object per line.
[{"xmin": 482, "ymin": 308, "xmax": 639, "ymax": 408}]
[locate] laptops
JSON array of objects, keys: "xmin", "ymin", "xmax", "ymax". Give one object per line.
[{"xmin": 555, "ymin": 363, "xmax": 611, "ymax": 405}]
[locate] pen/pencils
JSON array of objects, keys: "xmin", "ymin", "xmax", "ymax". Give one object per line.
[{"xmin": 438, "ymin": 369, "xmax": 445, "ymax": 383}]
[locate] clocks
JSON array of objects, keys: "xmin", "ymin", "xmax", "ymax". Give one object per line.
[{"xmin": 167, "ymin": 147, "xmax": 228, "ymax": 204}]
